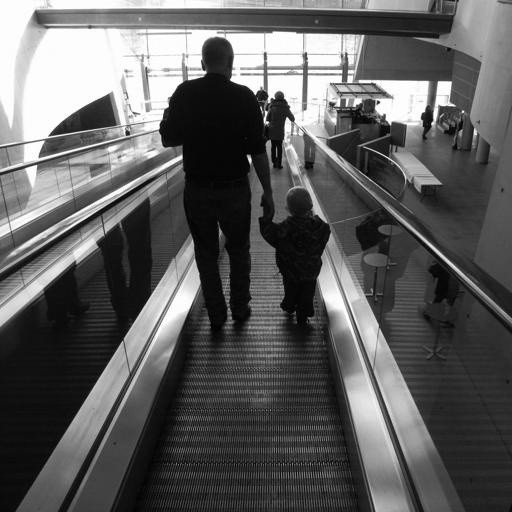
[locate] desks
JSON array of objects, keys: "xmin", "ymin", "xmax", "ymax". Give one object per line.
[{"xmin": 391, "ymin": 152, "xmax": 443, "ymax": 202}]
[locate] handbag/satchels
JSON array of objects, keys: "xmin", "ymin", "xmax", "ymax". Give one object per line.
[{"xmin": 263, "ymin": 124, "xmax": 271, "ymax": 140}]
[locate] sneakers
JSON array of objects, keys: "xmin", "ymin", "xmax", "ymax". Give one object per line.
[
  {"xmin": 210, "ymin": 314, "xmax": 226, "ymax": 331},
  {"xmin": 231, "ymin": 306, "xmax": 251, "ymax": 321}
]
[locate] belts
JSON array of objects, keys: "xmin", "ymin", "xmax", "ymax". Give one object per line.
[{"xmin": 185, "ymin": 175, "xmax": 249, "ymax": 190}]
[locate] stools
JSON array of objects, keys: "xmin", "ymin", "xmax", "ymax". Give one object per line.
[{"xmin": 363, "ymin": 224, "xmax": 452, "ymax": 362}]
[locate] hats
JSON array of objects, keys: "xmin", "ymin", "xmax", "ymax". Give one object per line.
[{"xmin": 286, "ymin": 188, "xmax": 313, "ymax": 210}]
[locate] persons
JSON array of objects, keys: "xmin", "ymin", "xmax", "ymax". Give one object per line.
[
  {"xmin": 159, "ymin": 36, "xmax": 275, "ymax": 334},
  {"xmin": 421, "ymin": 260, "xmax": 460, "ymax": 328},
  {"xmin": 258, "ymin": 187, "xmax": 331, "ymax": 330},
  {"xmin": 95, "ymin": 199, "xmax": 152, "ymax": 317},
  {"xmin": 266, "ymin": 91, "xmax": 295, "ymax": 169},
  {"xmin": 45, "ymin": 266, "xmax": 90, "ymax": 326},
  {"xmin": 256, "ymin": 87, "xmax": 268, "ymax": 112},
  {"xmin": 421, "ymin": 105, "xmax": 434, "ymax": 140},
  {"xmin": 355, "ymin": 207, "xmax": 415, "ymax": 315},
  {"xmin": 303, "ymin": 134, "xmax": 316, "ymax": 170}
]
